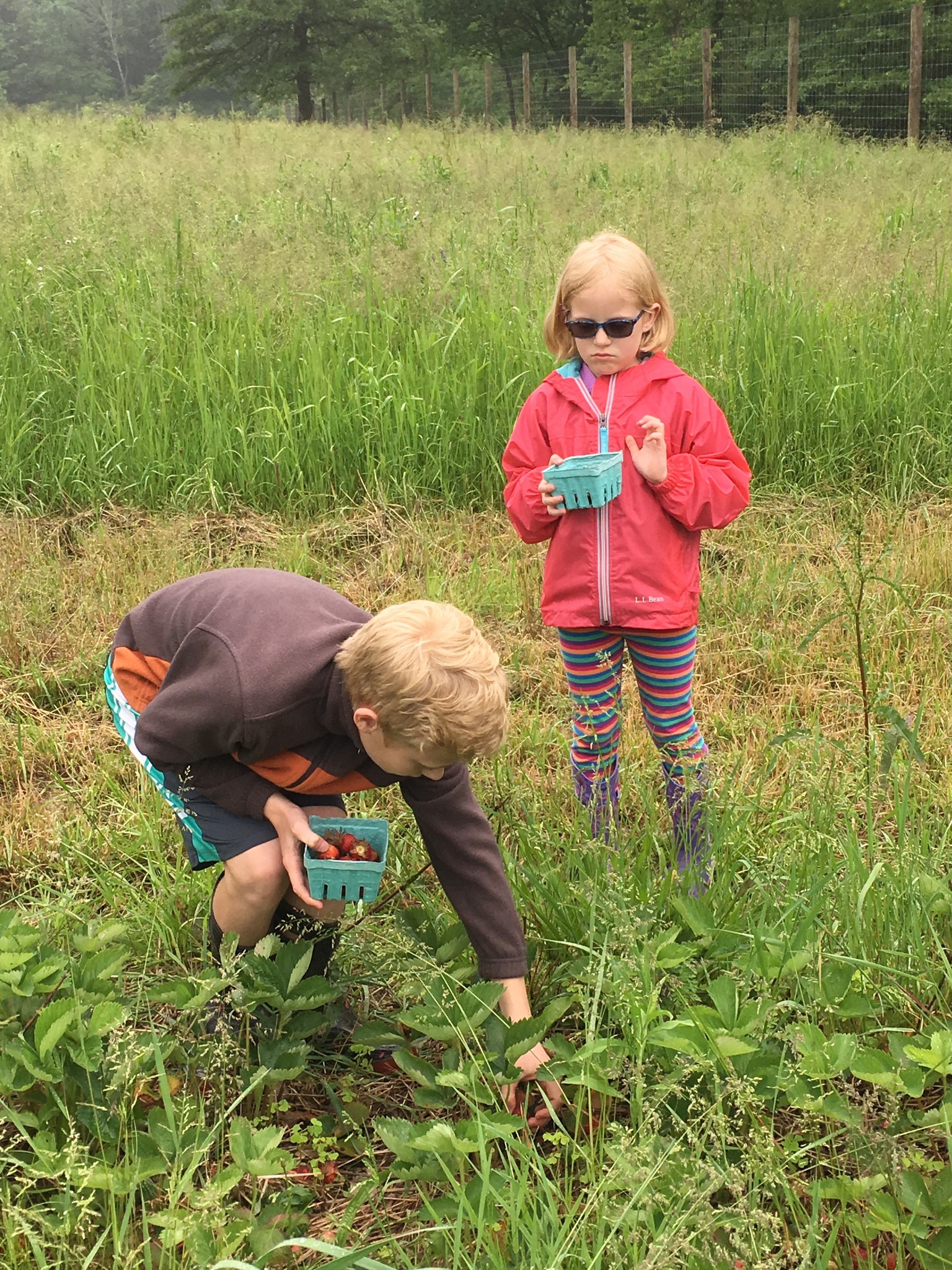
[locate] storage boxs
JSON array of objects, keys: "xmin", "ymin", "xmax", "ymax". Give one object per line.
[
  {"xmin": 540, "ymin": 446, "xmax": 629, "ymax": 510},
  {"xmin": 302, "ymin": 815, "xmax": 390, "ymax": 902}
]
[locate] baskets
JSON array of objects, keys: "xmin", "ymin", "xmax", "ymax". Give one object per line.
[
  {"xmin": 305, "ymin": 814, "xmax": 388, "ymax": 902},
  {"xmin": 541, "ymin": 450, "xmax": 623, "ymax": 510}
]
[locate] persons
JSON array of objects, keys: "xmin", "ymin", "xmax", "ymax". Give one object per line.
[
  {"xmin": 503, "ymin": 235, "xmax": 755, "ymax": 900},
  {"xmin": 99, "ymin": 567, "xmax": 564, "ymax": 1132}
]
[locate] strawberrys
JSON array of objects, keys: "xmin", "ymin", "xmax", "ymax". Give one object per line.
[{"xmin": 138, "ymin": 832, "xmax": 901, "ymax": 1270}]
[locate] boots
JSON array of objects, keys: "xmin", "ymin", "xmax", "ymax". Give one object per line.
[
  {"xmin": 569, "ymin": 749, "xmax": 619, "ymax": 871},
  {"xmin": 662, "ymin": 748, "xmax": 712, "ymax": 897},
  {"xmin": 267, "ymin": 897, "xmax": 394, "ymax": 1067},
  {"xmin": 209, "ymin": 869, "xmax": 270, "ymax": 1066}
]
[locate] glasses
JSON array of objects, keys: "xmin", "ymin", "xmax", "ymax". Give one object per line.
[{"xmin": 564, "ymin": 310, "xmax": 645, "ymax": 339}]
